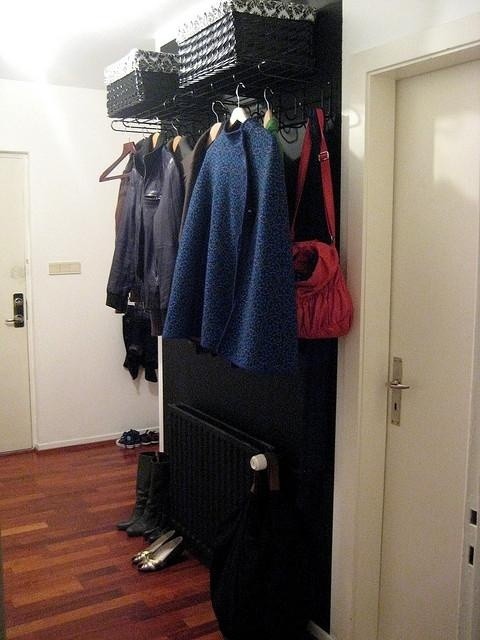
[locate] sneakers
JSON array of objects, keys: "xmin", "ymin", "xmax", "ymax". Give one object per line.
[
  {"xmin": 115, "ymin": 429, "xmax": 141, "ymax": 449},
  {"xmin": 140, "ymin": 431, "xmax": 159, "ymax": 445}
]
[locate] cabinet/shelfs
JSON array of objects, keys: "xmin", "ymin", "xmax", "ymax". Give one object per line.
[{"xmin": 102, "ymin": 0, "xmax": 343, "ymax": 640}]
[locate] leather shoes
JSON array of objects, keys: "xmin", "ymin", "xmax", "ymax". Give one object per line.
[{"xmin": 131, "ymin": 526, "xmax": 186, "ymax": 572}]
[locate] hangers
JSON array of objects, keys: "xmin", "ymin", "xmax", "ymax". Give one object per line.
[{"xmin": 98, "ymin": 82, "xmax": 274, "ymax": 182}]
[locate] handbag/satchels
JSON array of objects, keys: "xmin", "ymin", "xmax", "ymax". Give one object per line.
[
  {"xmin": 208, "ymin": 488, "xmax": 326, "ymax": 639},
  {"xmin": 291, "ymin": 240, "xmax": 352, "ymax": 339}
]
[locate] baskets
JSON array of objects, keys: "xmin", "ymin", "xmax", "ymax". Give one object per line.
[
  {"xmin": 176, "ymin": 1, "xmax": 318, "ymax": 90},
  {"xmin": 106, "ymin": 49, "xmax": 179, "ymax": 117}
]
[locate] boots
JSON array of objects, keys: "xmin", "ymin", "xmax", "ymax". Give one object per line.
[
  {"xmin": 117, "ymin": 452, "xmax": 169, "ymax": 530},
  {"xmin": 126, "ymin": 454, "xmax": 169, "ymax": 536}
]
